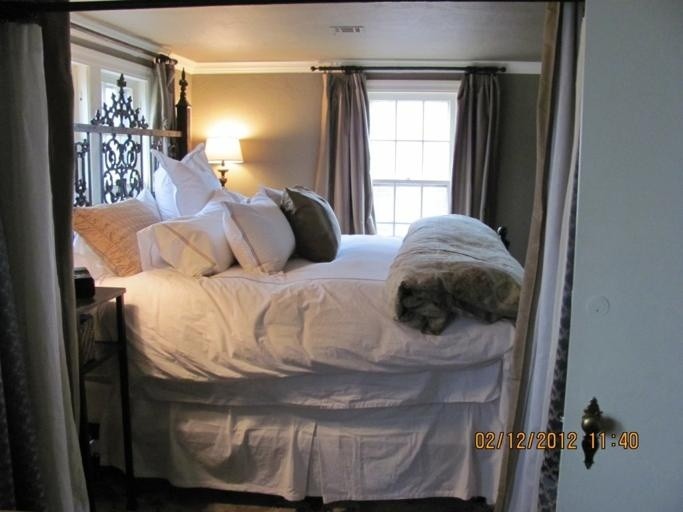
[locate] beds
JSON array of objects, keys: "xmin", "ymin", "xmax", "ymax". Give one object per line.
[{"xmin": 71, "ymin": 68, "xmax": 517, "ymax": 498}]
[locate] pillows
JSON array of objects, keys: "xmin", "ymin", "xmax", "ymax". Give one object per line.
[{"xmin": 72, "ymin": 140, "xmax": 343, "ymax": 286}]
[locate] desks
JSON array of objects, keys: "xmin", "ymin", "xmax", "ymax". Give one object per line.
[{"xmin": 69, "ymin": 285, "xmax": 139, "ymax": 512}]
